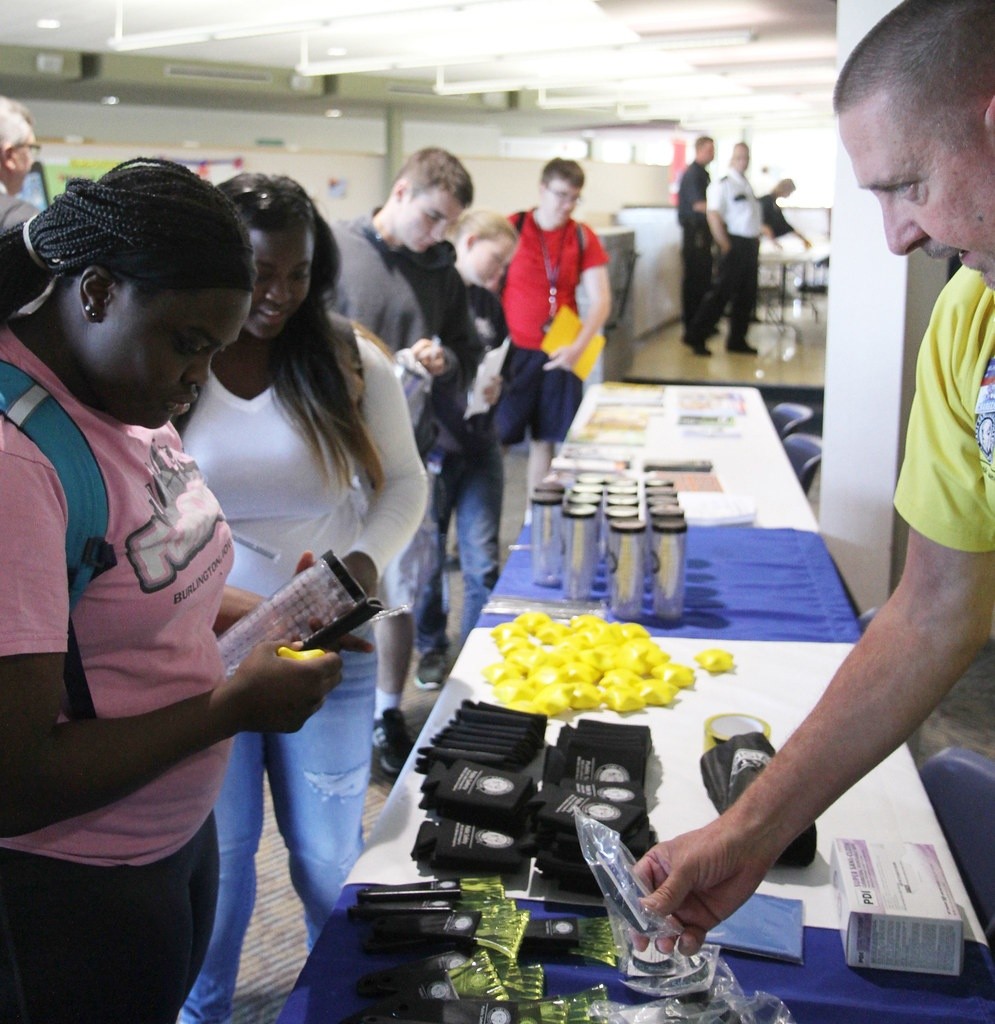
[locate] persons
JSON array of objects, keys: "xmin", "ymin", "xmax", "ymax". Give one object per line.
[
  {"xmin": 0, "ymin": 96, "xmax": 44, "ymax": 232},
  {"xmin": 324, "ymin": 149, "xmax": 473, "ymax": 773},
  {"xmin": 0, "ymin": 152, "xmax": 377, "ymax": 1024},
  {"xmin": 624, "ymin": 0, "xmax": 995, "ymax": 955},
  {"xmin": 177, "ymin": 170, "xmax": 430, "ymax": 1024},
  {"xmin": 413, "ymin": 210, "xmax": 521, "ymax": 690},
  {"xmin": 677, "ymin": 136, "xmax": 809, "ymax": 356},
  {"xmin": 448, "ymin": 159, "xmax": 612, "ymax": 567}
]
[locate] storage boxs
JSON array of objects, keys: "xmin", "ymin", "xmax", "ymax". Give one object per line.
[{"xmin": 830, "ymin": 837, "xmax": 964, "ymax": 979}]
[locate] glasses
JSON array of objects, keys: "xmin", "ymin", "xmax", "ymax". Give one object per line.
[
  {"xmin": 231, "ymin": 190, "xmax": 317, "ymax": 219},
  {"xmin": 12, "ymin": 145, "xmax": 40, "ymax": 155}
]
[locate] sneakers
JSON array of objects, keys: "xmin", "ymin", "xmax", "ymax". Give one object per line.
[
  {"xmin": 414, "ymin": 642, "xmax": 448, "ymax": 688},
  {"xmin": 373, "ymin": 708, "xmax": 414, "ymax": 773}
]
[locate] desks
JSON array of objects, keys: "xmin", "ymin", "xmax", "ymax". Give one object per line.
[
  {"xmin": 338, "ymin": 374, "xmax": 995, "ymax": 1024},
  {"xmin": 756, "ymin": 253, "xmax": 820, "ymax": 327}
]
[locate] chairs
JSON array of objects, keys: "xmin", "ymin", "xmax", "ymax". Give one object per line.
[
  {"xmin": 916, "ymin": 745, "xmax": 995, "ymax": 962},
  {"xmin": 769, "ymin": 400, "xmax": 813, "ymax": 439},
  {"xmin": 782, "ymin": 432, "xmax": 821, "ymax": 496}
]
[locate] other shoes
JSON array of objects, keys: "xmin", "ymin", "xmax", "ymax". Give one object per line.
[
  {"xmin": 681, "ymin": 332, "xmax": 714, "ymax": 357},
  {"xmin": 726, "ymin": 339, "xmax": 757, "ymax": 354}
]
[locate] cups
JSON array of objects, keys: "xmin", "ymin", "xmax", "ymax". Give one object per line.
[
  {"xmin": 530, "ymin": 477, "xmax": 689, "ymax": 627},
  {"xmin": 213, "ymin": 549, "xmax": 366, "ymax": 684}
]
